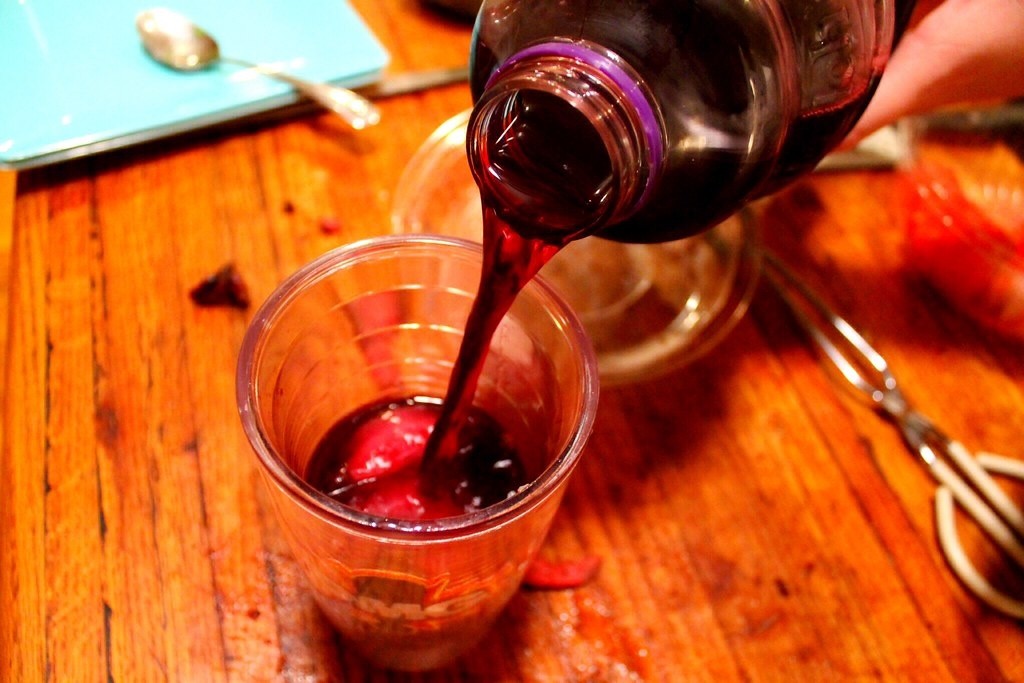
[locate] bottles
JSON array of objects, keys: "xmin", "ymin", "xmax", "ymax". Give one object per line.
[{"xmin": 466, "ymin": 0, "xmax": 918, "ymax": 243}]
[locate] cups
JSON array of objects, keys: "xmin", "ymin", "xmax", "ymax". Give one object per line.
[{"xmin": 235, "ymin": 234, "xmax": 599, "ymax": 670}]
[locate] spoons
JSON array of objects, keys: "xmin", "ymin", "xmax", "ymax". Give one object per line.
[{"xmin": 137, "ymin": 12, "xmax": 383, "ymax": 131}]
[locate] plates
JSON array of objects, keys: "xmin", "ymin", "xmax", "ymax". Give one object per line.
[{"xmin": 393, "ymin": 104, "xmax": 764, "ymax": 387}]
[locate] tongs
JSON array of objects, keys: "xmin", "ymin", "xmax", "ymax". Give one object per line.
[{"xmin": 756, "ymin": 251, "xmax": 1024, "ymax": 619}]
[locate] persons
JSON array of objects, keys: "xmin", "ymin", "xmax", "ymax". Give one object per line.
[{"xmin": 831, "ymin": 0, "xmax": 1023, "ymax": 154}]
